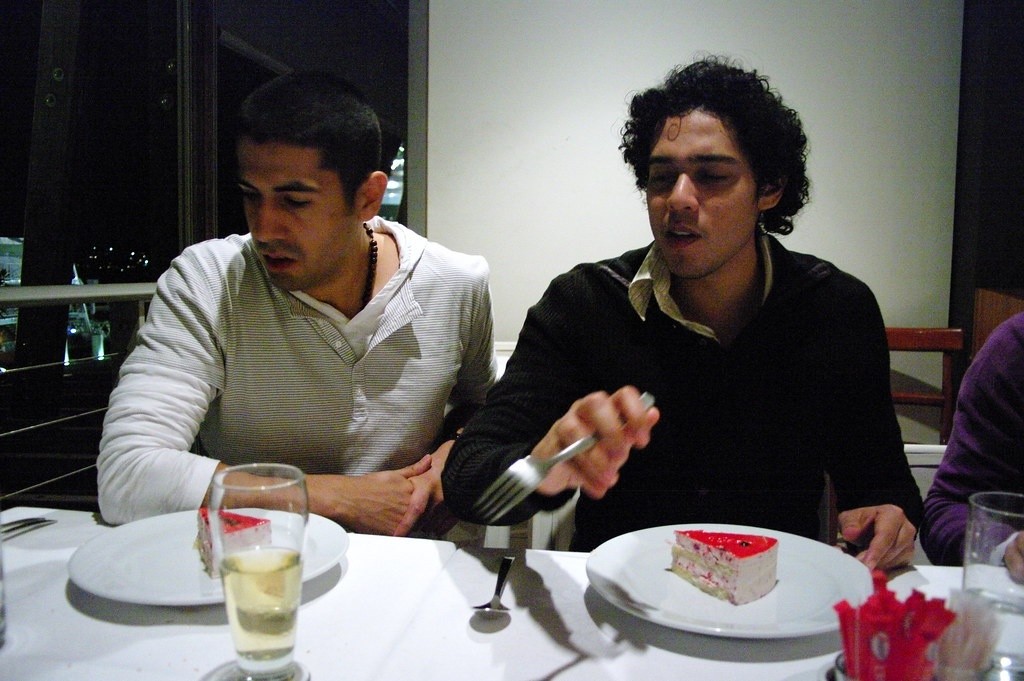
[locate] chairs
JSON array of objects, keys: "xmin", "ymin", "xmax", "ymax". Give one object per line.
[{"xmin": 886, "ymin": 324, "xmax": 963, "ymax": 446}]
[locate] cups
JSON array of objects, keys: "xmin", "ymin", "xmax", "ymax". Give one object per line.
[
  {"xmin": 963, "ymin": 492, "xmax": 1024, "ymax": 681},
  {"xmin": 209, "ymin": 462, "xmax": 310, "ymax": 681}
]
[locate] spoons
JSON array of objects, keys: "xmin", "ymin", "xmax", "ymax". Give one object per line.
[{"xmin": 473, "ymin": 556, "xmax": 515, "ymax": 620}]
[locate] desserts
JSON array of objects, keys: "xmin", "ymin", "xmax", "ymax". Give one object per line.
[
  {"xmin": 197, "ymin": 508, "xmax": 272, "ymax": 577},
  {"xmin": 671, "ymin": 526, "xmax": 778, "ymax": 604}
]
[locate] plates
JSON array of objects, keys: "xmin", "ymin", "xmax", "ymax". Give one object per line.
[
  {"xmin": 586, "ymin": 523, "xmax": 875, "ymax": 638},
  {"xmin": 67, "ymin": 507, "xmax": 349, "ymax": 606}
]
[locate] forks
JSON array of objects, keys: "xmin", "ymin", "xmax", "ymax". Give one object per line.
[{"xmin": 471, "ymin": 391, "xmax": 655, "ymax": 524}]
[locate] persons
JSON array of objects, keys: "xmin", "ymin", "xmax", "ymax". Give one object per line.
[
  {"xmin": 96, "ymin": 76, "xmax": 498, "ymax": 540},
  {"xmin": 442, "ymin": 55, "xmax": 926, "ymax": 573},
  {"xmin": 920, "ymin": 310, "xmax": 1024, "ymax": 581}
]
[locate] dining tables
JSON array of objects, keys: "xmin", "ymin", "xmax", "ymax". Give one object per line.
[{"xmin": 0, "ymin": 507, "xmax": 1024, "ymax": 681}]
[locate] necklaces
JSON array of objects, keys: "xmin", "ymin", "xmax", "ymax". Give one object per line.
[{"xmin": 362, "ymin": 221, "xmax": 379, "ymax": 311}]
